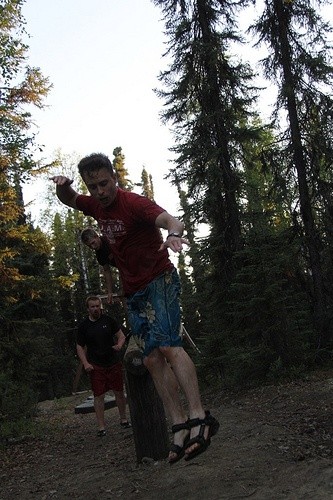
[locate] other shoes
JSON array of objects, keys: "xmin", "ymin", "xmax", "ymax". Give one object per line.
[
  {"xmin": 97, "ymin": 427, "xmax": 106, "ymax": 436},
  {"xmin": 120, "ymin": 419, "xmax": 132, "ymax": 429}
]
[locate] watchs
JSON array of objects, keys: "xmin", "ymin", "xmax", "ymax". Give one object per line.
[{"xmin": 167, "ymin": 232, "xmax": 181, "ymax": 240}]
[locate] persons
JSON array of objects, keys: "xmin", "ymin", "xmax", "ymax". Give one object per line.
[
  {"xmin": 49, "ymin": 153, "xmax": 220, "ymax": 464},
  {"xmin": 76, "ymin": 296, "xmax": 130, "ymax": 436},
  {"xmin": 82, "ymin": 229, "xmax": 121, "ymax": 305}
]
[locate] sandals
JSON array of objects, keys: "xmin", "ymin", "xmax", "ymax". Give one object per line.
[
  {"xmin": 183, "ymin": 410, "xmax": 220, "ymax": 461},
  {"xmin": 167, "ymin": 414, "xmax": 191, "ymax": 465}
]
